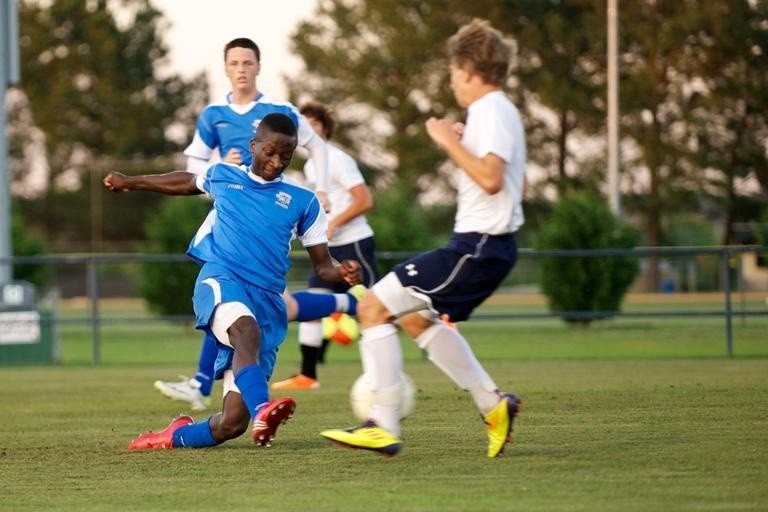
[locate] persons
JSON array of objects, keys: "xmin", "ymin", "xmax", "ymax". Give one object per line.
[
  {"xmin": 316, "ymin": 15, "xmax": 528, "ymax": 460},
  {"xmin": 101, "ymin": 112, "xmax": 364, "ymax": 449},
  {"xmin": 266, "ymin": 104, "xmax": 459, "ymax": 388},
  {"xmin": 152, "ymin": 37, "xmax": 333, "ymax": 416}
]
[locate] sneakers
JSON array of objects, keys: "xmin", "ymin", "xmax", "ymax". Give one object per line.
[
  {"xmin": 250, "ymin": 395, "xmax": 298, "ymax": 449},
  {"xmin": 318, "ymin": 417, "xmax": 403, "ymax": 458},
  {"xmin": 481, "ymin": 390, "xmax": 523, "ymax": 460},
  {"xmin": 269, "ymin": 373, "xmax": 321, "ymax": 391},
  {"xmin": 125, "ymin": 411, "xmax": 195, "ymax": 451},
  {"xmin": 151, "ymin": 378, "xmax": 213, "ymax": 413}
]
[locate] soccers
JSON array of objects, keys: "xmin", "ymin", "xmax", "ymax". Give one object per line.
[{"xmin": 350, "ymin": 370, "xmax": 415, "ymax": 422}]
